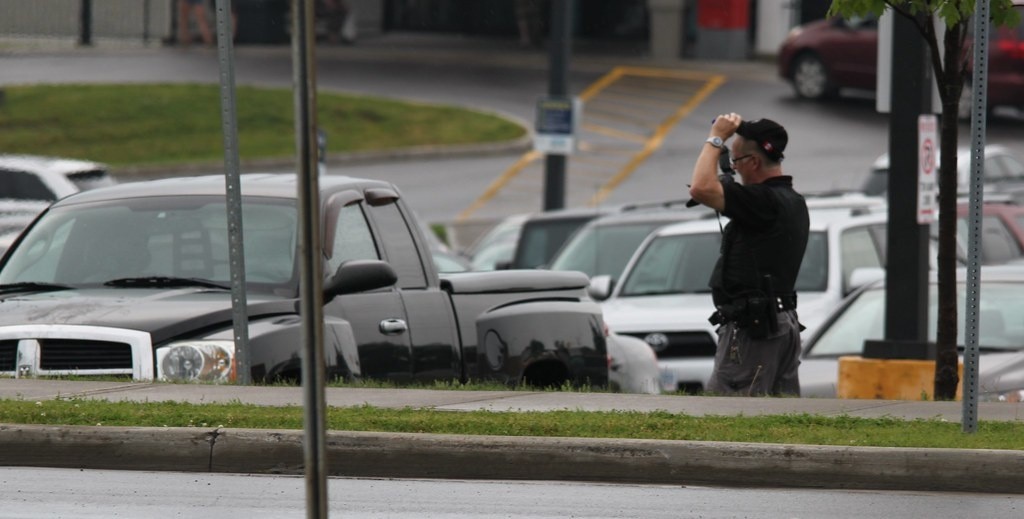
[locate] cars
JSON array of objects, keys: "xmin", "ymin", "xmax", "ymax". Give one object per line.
[
  {"xmin": 591, "ymin": 205, "xmax": 886, "ymax": 394},
  {"xmin": 464, "ymin": 151, "xmax": 1024, "ymax": 270},
  {"xmin": 778, "ymin": 1, "xmax": 1024, "ymax": 122},
  {"xmin": 1, "ymin": 157, "xmax": 126, "ymax": 255},
  {"xmin": 795, "ymin": 271, "xmax": 1024, "ymax": 402}
]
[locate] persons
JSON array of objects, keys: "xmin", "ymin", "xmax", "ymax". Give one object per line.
[{"xmin": 689, "ymin": 112, "xmax": 810, "ymax": 397}]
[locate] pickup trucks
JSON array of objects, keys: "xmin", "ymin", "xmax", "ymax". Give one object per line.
[{"xmin": 1, "ymin": 171, "xmax": 609, "ymax": 406}]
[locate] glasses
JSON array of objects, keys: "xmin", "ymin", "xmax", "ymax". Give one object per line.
[{"xmin": 732, "ymin": 153, "xmax": 752, "ymax": 163}]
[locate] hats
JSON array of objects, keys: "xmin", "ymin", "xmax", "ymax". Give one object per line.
[{"xmin": 735, "ymin": 119, "xmax": 788, "ymax": 162}]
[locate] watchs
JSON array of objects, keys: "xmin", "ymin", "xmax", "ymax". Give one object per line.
[{"xmin": 706, "ymin": 137, "xmax": 724, "ymax": 150}]
